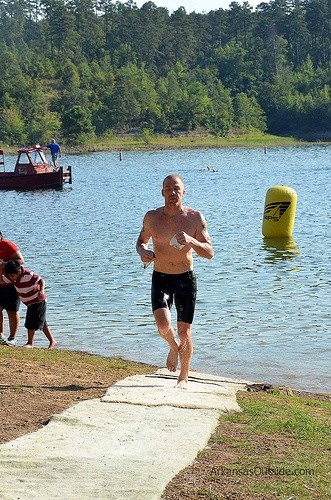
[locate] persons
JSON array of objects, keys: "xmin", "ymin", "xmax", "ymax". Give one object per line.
[
  {"xmin": 42, "ymin": 138, "xmax": 62, "ymax": 171},
  {"xmin": 136, "ymin": 174, "xmax": 213, "ymax": 390},
  {"xmin": 0, "ymin": 231, "xmax": 24, "ymax": 346},
  {"xmin": 4, "ymin": 259, "xmax": 57, "ymax": 349}
]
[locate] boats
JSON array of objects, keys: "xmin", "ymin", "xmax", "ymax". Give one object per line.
[{"xmin": 0, "ymin": 142, "xmax": 73, "ymax": 192}]
